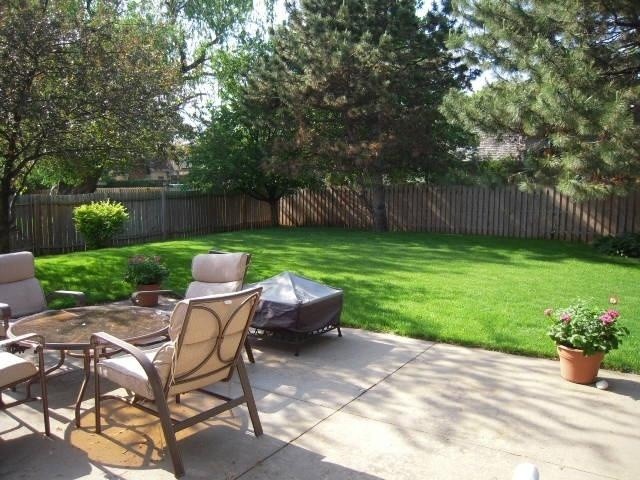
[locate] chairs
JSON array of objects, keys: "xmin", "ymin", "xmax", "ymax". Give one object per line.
[
  {"xmin": 0, "ymin": 251, "xmax": 85, "ymax": 392},
  {"xmin": 90, "ymin": 286, "xmax": 264, "ymax": 478},
  {"xmin": 185, "ymin": 251, "xmax": 256, "ymax": 365},
  {"xmin": 0, "ymin": 325, "xmax": 51, "ymax": 435}
]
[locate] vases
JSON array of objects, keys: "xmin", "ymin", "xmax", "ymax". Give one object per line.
[
  {"xmin": 135, "ymin": 284, "xmax": 159, "ymax": 307},
  {"xmin": 553, "ymin": 340, "xmax": 604, "ymax": 384}
]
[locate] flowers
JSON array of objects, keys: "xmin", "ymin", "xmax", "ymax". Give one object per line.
[
  {"xmin": 545, "ymin": 296, "xmax": 630, "ymax": 358},
  {"xmin": 123, "ymin": 255, "xmax": 170, "ymax": 287}
]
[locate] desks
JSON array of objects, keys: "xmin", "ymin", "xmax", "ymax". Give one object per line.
[{"xmin": 6, "ymin": 306, "xmax": 172, "ymax": 427}]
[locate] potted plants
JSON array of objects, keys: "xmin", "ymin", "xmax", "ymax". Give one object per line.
[{"xmin": 72, "ymin": 197, "xmax": 131, "ymax": 250}]
